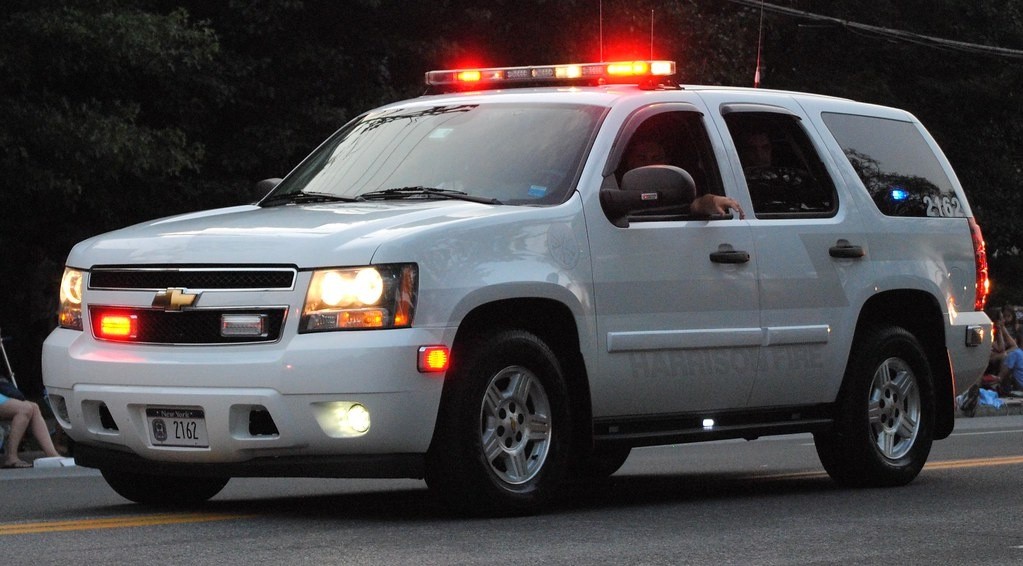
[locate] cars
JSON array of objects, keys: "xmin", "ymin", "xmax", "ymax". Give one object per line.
[{"xmin": 39, "ymin": 62, "xmax": 996, "ymax": 517}]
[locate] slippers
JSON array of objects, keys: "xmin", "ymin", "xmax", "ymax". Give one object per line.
[{"xmin": 2, "ymin": 459, "xmax": 33, "ymax": 468}]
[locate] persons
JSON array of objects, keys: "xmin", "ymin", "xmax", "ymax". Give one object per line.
[
  {"xmin": 986, "ymin": 305, "xmax": 1023, "ymax": 391},
  {"xmin": 0, "ymin": 338, "xmax": 62, "ymax": 468},
  {"xmin": 628, "ymin": 138, "xmax": 746, "ymax": 220}
]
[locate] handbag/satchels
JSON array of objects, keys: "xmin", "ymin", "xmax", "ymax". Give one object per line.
[{"xmin": 0, "ymin": 382, "xmax": 25, "ymax": 401}]
